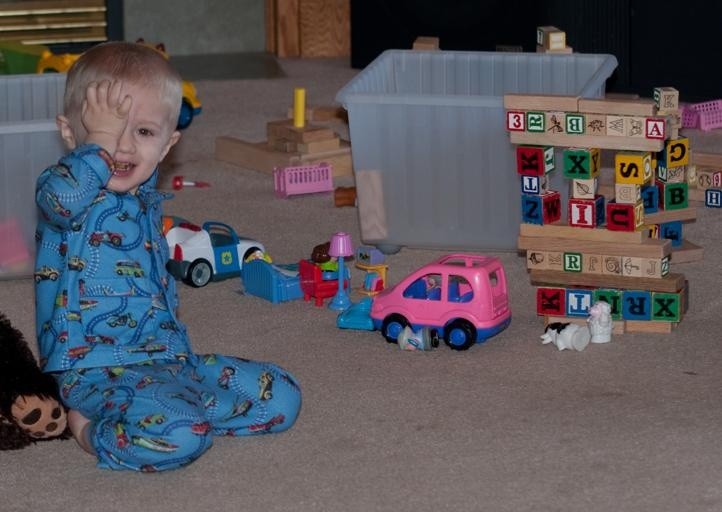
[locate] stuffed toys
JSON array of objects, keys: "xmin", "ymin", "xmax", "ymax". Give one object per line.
[{"xmin": 1, "ymin": 312, "xmax": 67, "ymax": 452}]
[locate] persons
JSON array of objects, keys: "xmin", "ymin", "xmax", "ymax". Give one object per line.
[{"xmin": 34, "ymin": 42, "xmax": 301, "ymax": 471}]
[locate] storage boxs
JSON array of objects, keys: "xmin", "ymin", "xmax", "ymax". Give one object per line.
[
  {"xmin": 334, "ymin": 46, "xmax": 621, "ymax": 250},
  {"xmin": 1, "ymin": 72, "xmax": 71, "ymax": 281}
]
